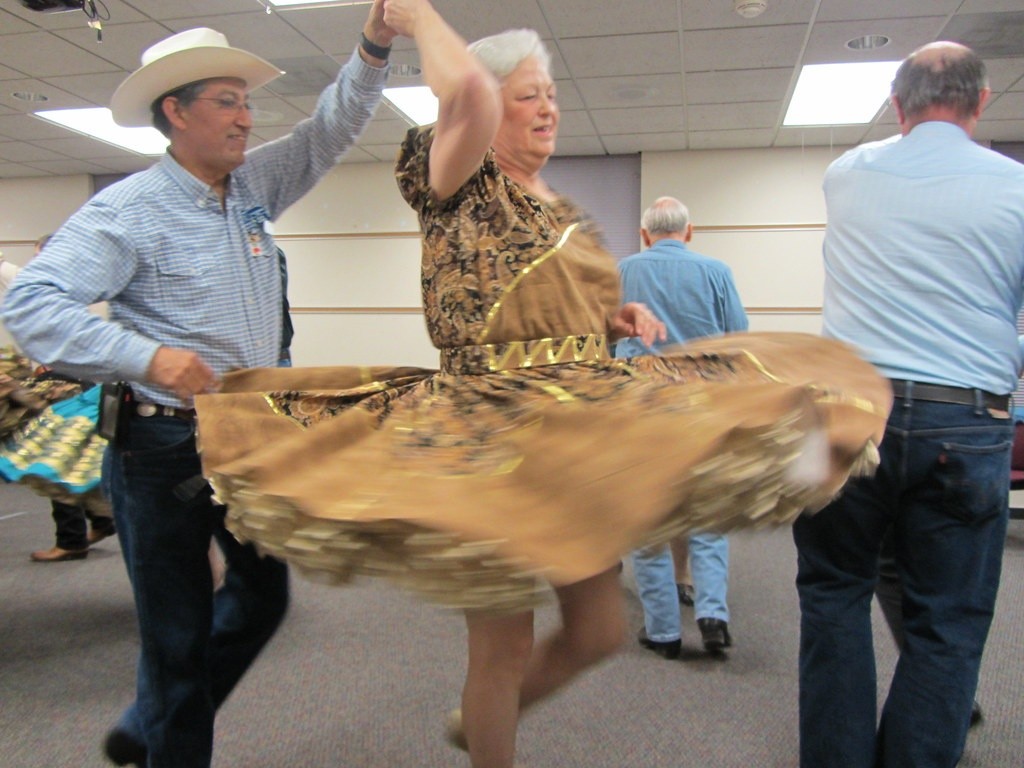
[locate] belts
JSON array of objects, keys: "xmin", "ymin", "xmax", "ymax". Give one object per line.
[
  {"xmin": 136, "ymin": 403, "xmax": 196, "ymax": 422},
  {"xmin": 892, "ymin": 378, "xmax": 1010, "ymax": 410}
]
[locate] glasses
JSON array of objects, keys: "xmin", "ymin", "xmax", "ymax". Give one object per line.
[{"xmin": 193, "ymin": 97, "xmax": 254, "ymax": 114}]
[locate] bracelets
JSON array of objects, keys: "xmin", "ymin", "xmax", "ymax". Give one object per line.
[{"xmin": 359, "ymin": 31, "xmax": 393, "ymax": 61}]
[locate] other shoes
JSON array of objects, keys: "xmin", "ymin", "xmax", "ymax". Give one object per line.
[
  {"xmin": 445, "ymin": 709, "xmax": 468, "ymax": 748},
  {"xmin": 970, "ymin": 699, "xmax": 982, "ymax": 726},
  {"xmin": 637, "ymin": 626, "xmax": 681, "ymax": 659},
  {"xmin": 677, "ymin": 584, "xmax": 694, "ymax": 606},
  {"xmin": 103, "ymin": 728, "xmax": 134, "ymax": 768},
  {"xmin": 696, "ymin": 618, "xmax": 733, "ymax": 647}
]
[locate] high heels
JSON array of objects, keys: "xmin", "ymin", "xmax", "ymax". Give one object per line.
[
  {"xmin": 30, "ymin": 546, "xmax": 89, "ymax": 561},
  {"xmin": 87, "ymin": 524, "xmax": 115, "ymax": 543}
]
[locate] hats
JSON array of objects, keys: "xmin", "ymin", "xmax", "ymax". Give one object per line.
[{"xmin": 109, "ymin": 28, "xmax": 287, "ymax": 128}]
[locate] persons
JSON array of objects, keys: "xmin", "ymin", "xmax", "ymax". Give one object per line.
[
  {"xmin": 374, "ymin": 1, "xmax": 667, "ymax": 768},
  {"xmin": 1, "ymin": 0, "xmax": 404, "ymax": 768},
  {"xmin": 609, "ymin": 195, "xmax": 749, "ymax": 656},
  {"xmin": 669, "ymin": 536, "xmax": 698, "ymax": 605},
  {"xmin": 791, "ymin": 39, "xmax": 1024, "ymax": 768},
  {"xmin": 0, "ymin": 233, "xmax": 121, "ymax": 561}
]
